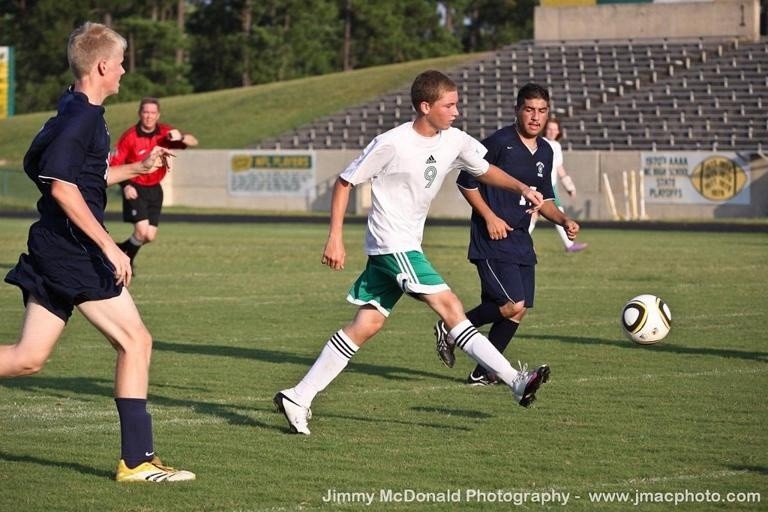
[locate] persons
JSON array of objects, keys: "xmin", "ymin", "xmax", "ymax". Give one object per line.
[
  {"xmin": 528, "ymin": 115, "xmax": 589, "ymax": 253},
  {"xmin": 435, "ymin": 2, "xmax": 497, "ymax": 53},
  {"xmin": 272, "ymin": 69, "xmax": 549, "ymax": 435},
  {"xmin": 101, "ymin": 99, "xmax": 199, "ymax": 274},
  {"xmin": 1, "ymin": 21, "xmax": 194, "ymax": 482},
  {"xmin": 434, "ymin": 83, "xmax": 580, "ymax": 387}
]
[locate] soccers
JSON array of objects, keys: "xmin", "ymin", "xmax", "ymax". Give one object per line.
[{"xmin": 623, "ymin": 293, "xmax": 674, "ymax": 344}]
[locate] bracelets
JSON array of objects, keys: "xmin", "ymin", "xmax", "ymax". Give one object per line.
[{"xmin": 180, "ymin": 131, "xmax": 186, "ymax": 143}]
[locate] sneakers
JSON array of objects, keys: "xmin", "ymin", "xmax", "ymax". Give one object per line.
[
  {"xmin": 274, "ymin": 386, "xmax": 312, "ymax": 435},
  {"xmin": 565, "ymin": 242, "xmax": 588, "ymax": 253},
  {"xmin": 433, "ymin": 320, "xmax": 455, "ymax": 369},
  {"xmin": 116, "ymin": 455, "xmax": 196, "ymax": 482},
  {"xmin": 467, "ymin": 372, "xmax": 505, "ymax": 387},
  {"xmin": 513, "ymin": 363, "xmax": 551, "ymax": 407}
]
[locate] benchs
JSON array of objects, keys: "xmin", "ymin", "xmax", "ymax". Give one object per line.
[{"xmin": 242, "ymin": 36, "xmax": 768, "ymax": 161}]
